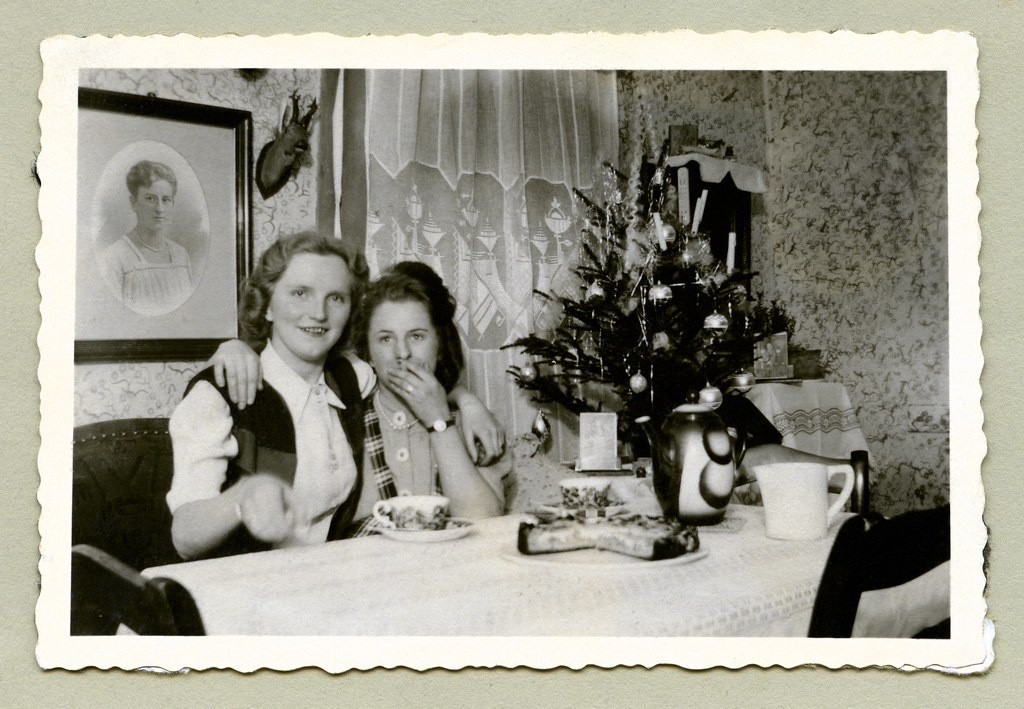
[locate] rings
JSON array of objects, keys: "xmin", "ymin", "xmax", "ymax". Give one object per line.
[{"xmin": 405, "ymin": 385, "xmax": 414, "ymax": 392}]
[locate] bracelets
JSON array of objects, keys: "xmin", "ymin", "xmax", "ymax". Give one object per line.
[{"xmin": 234, "ymin": 477, "xmax": 245, "ymax": 518}]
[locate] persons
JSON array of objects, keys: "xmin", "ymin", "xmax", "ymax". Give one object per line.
[
  {"xmin": 97, "ymin": 161, "xmax": 192, "ymax": 315},
  {"xmin": 209, "ymin": 261, "xmax": 512, "ymax": 544},
  {"xmin": 156, "ymin": 230, "xmax": 507, "ymax": 566}
]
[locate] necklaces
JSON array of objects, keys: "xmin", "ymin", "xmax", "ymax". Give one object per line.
[
  {"xmin": 377, "ymin": 388, "xmax": 420, "ymax": 430},
  {"xmin": 132, "ymin": 230, "xmax": 166, "ymax": 252}
]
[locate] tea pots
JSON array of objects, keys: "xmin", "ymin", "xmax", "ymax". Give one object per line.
[{"xmin": 635, "ymin": 392, "xmax": 748, "ymax": 526}]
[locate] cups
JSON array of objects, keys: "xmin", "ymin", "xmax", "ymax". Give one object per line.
[
  {"xmin": 753, "ymin": 463, "xmax": 855, "ymax": 541},
  {"xmin": 374, "ymin": 496, "xmax": 450, "ymax": 531},
  {"xmin": 559, "ymin": 479, "xmax": 613, "ymax": 509}
]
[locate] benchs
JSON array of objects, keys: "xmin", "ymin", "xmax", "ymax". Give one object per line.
[{"xmin": 72, "ymin": 418, "xmax": 276, "ymax": 575}]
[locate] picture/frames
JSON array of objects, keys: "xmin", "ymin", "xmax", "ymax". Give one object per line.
[{"xmin": 74, "ymin": 87, "xmax": 254, "ymax": 362}]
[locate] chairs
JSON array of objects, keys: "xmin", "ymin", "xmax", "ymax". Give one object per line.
[
  {"xmin": 70, "ymin": 544, "xmax": 206, "ymax": 636},
  {"xmin": 806, "ymin": 501, "xmax": 951, "ymax": 640}
]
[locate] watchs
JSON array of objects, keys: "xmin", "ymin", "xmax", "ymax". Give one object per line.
[{"xmin": 426, "ymin": 417, "xmax": 455, "ymax": 433}]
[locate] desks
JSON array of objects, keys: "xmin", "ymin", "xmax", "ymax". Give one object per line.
[
  {"xmin": 725, "ymin": 377, "xmax": 880, "ymax": 513},
  {"xmin": 115, "ymin": 503, "xmax": 890, "ymax": 637}
]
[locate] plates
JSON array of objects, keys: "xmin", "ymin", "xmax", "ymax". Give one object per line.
[
  {"xmin": 542, "ymin": 500, "xmax": 627, "ymax": 521},
  {"xmin": 369, "ymin": 517, "xmax": 474, "ymax": 542}
]
